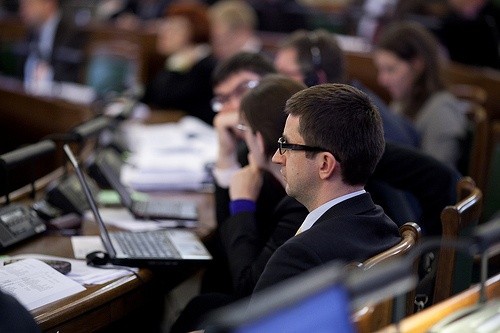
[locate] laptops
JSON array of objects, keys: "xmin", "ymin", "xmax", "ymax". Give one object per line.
[
  {"xmin": 64, "ymin": 145, "xmax": 213, "ymax": 263},
  {"xmin": 205, "ymin": 259, "xmax": 357, "ymax": 333}
]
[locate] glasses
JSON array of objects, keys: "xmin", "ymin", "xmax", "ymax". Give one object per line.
[
  {"xmin": 212, "ymin": 80, "xmax": 260, "ymax": 112},
  {"xmin": 236, "ymin": 122, "xmax": 249, "ymax": 132},
  {"xmin": 277, "ymin": 137, "xmax": 340, "ymax": 163},
  {"xmin": 274, "ymin": 64, "xmax": 303, "ymax": 77}
]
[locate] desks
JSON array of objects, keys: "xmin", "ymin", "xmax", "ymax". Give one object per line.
[{"xmin": 0, "ymin": 74, "xmax": 218, "ymax": 333}]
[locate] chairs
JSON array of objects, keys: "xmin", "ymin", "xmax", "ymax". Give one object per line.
[
  {"xmin": 434, "ymin": 175, "xmax": 483, "ymax": 301},
  {"xmin": 342, "ymin": 222, "xmax": 422, "ymax": 333},
  {"xmin": 456, "ymin": 83, "xmax": 490, "ymax": 192}
]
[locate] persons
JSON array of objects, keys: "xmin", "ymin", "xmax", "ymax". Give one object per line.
[{"xmin": 0, "ymin": 0, "xmax": 500, "ymax": 333}]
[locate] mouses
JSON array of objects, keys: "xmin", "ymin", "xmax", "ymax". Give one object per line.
[{"xmin": 86, "ymin": 251, "xmax": 110, "ymax": 265}]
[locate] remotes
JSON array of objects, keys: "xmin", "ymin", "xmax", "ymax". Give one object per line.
[{"xmin": 4, "ymin": 258, "xmax": 71, "ymax": 274}]
